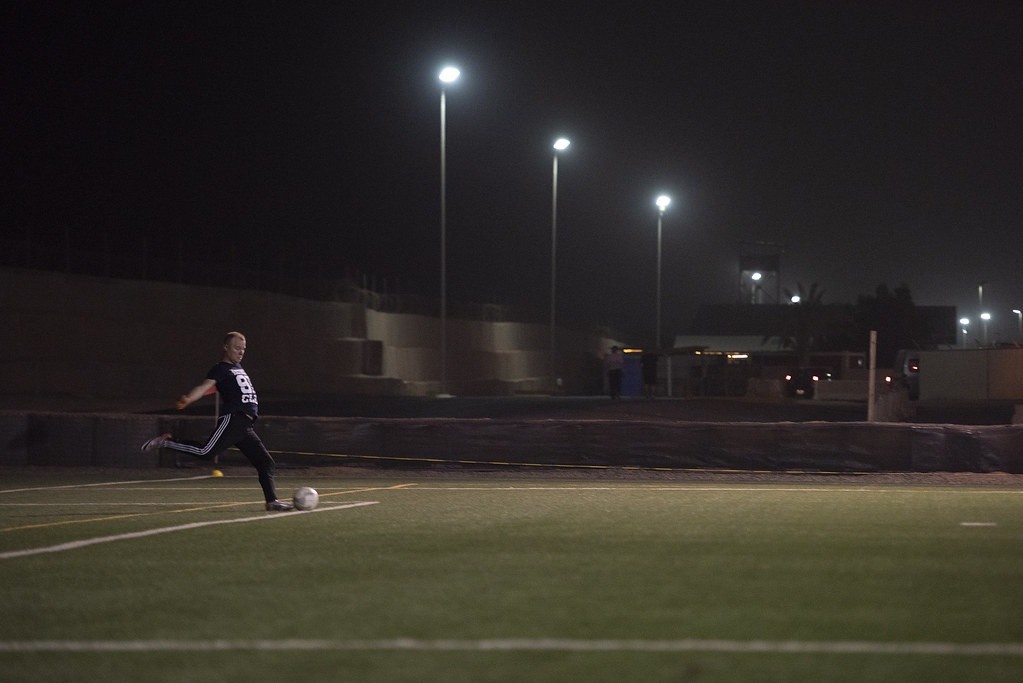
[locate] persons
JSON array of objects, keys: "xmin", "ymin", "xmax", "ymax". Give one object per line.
[
  {"xmin": 141, "ymin": 332, "xmax": 294, "ymax": 511},
  {"xmin": 606, "ymin": 345, "xmax": 625, "ymax": 400}
]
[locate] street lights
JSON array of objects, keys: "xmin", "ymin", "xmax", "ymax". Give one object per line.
[
  {"xmin": 980, "ymin": 313, "xmax": 992, "ymax": 346},
  {"xmin": 1012, "ymin": 309, "xmax": 1023, "ymax": 347},
  {"xmin": 548, "ymin": 135, "xmax": 572, "ymax": 356},
  {"xmin": 652, "ymin": 193, "xmax": 672, "ymax": 351},
  {"xmin": 959, "ymin": 318, "xmax": 970, "ymax": 350},
  {"xmin": 434, "ymin": 63, "xmax": 465, "ymax": 392}
]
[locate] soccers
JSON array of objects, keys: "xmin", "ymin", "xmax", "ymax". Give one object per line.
[{"xmin": 293, "ymin": 486, "xmax": 320, "ymax": 511}]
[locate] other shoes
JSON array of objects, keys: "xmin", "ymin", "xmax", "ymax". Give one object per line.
[
  {"xmin": 266, "ymin": 500, "xmax": 293, "ymax": 510},
  {"xmin": 141, "ymin": 433, "xmax": 173, "ymax": 451}
]
